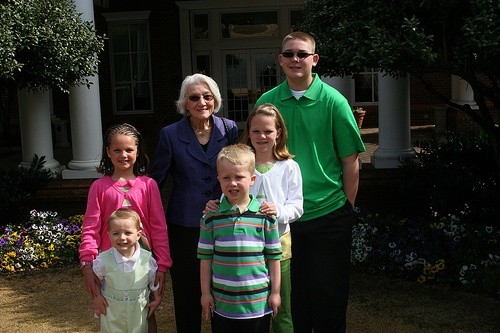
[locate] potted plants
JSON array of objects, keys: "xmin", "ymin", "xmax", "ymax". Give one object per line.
[{"xmin": 353, "ymin": 107, "xmax": 365, "ymax": 129}]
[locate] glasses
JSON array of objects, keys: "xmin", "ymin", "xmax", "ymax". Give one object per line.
[
  {"xmin": 280, "ymin": 51, "xmax": 314, "ymax": 58},
  {"xmin": 188, "ymin": 95, "xmax": 215, "ymax": 101}
]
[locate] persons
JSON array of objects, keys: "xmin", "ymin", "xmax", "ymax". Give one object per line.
[
  {"xmin": 90, "ymin": 207, "xmax": 161, "ymax": 333},
  {"xmin": 203, "ymin": 103, "xmax": 304, "ymax": 333},
  {"xmin": 147, "ymin": 74, "xmax": 238, "ymax": 333},
  {"xmin": 255, "ymin": 31, "xmax": 366, "ymax": 333},
  {"xmin": 197, "ymin": 143, "xmax": 283, "ymax": 333},
  {"xmin": 79, "ymin": 123, "xmax": 173, "ymax": 333}
]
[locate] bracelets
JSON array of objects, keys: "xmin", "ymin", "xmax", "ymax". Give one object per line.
[{"xmin": 80, "ymin": 261, "xmax": 92, "ymax": 269}]
